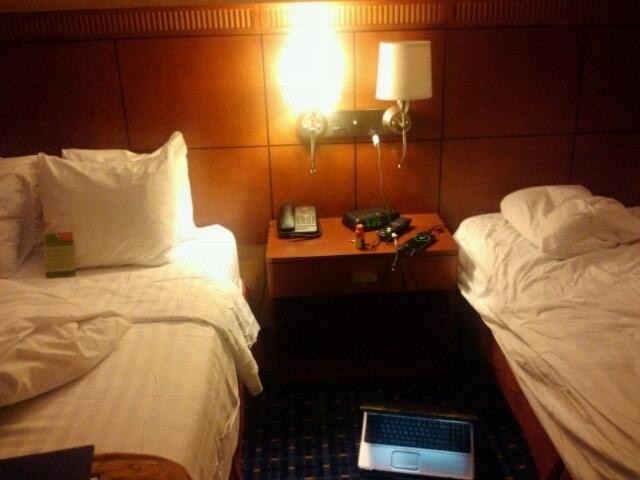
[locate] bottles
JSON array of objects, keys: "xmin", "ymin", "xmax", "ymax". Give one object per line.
[{"xmin": 355, "ymin": 224, "xmax": 365, "ymax": 250}]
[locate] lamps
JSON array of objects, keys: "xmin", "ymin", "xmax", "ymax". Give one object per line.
[
  {"xmin": 374, "ymin": 40, "xmax": 434, "ymax": 169},
  {"xmin": 281, "ymin": 39, "xmax": 346, "ymax": 175}
]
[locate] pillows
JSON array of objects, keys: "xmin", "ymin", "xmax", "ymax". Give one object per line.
[
  {"xmin": 60, "ymin": 130, "xmax": 201, "ymax": 242},
  {"xmin": 36, "ymin": 142, "xmax": 177, "ymax": 267},
  {"xmin": 0, "ymin": 153, "xmax": 38, "ymax": 278}
]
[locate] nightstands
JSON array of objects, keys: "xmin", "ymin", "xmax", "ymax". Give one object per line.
[{"xmin": 265, "ymin": 212, "xmax": 460, "ymax": 369}]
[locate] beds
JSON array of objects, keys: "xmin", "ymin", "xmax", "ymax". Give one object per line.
[
  {"xmin": 452, "ymin": 203, "xmax": 640, "ymax": 480},
  {"xmin": 0, "ymin": 222, "xmax": 264, "ymax": 480}
]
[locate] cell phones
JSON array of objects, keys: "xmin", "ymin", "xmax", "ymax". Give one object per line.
[{"xmin": 403, "ymin": 229, "xmax": 436, "ymax": 253}]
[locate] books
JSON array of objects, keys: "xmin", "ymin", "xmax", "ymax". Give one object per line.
[{"xmin": 42, "ymin": 231, "xmax": 76, "ymax": 278}]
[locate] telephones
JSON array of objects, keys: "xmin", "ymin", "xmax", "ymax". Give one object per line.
[{"xmin": 280, "ymin": 203, "xmax": 322, "ymax": 237}]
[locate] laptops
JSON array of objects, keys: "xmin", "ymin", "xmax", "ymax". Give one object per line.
[{"xmin": 355, "ymin": 398, "xmax": 484, "ymax": 479}]
